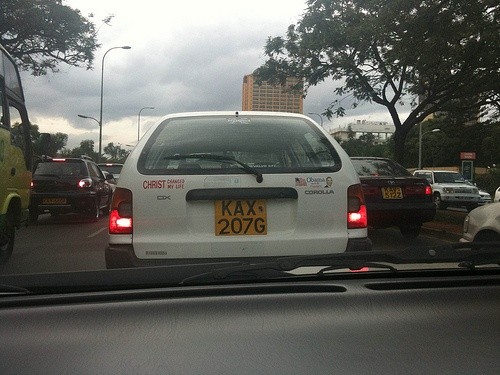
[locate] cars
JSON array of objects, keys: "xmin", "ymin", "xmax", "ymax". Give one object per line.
[
  {"xmin": 104, "ymin": 111, "xmax": 370, "ymax": 269},
  {"xmin": 493, "ymin": 187, "xmax": 500, "ymax": 202},
  {"xmin": 465, "ymin": 179, "xmax": 492, "ymax": 203},
  {"xmin": 138, "ymin": 106, "xmax": 156, "ymax": 141},
  {"xmin": 459, "ymin": 202, "xmax": 500, "ymax": 265},
  {"xmin": 348, "ymin": 156, "xmax": 435, "ymax": 238}
]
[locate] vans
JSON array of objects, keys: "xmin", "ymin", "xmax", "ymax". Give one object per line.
[{"xmin": 97, "ymin": 163, "xmax": 124, "ymax": 184}]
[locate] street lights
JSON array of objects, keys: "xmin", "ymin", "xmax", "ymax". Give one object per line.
[{"xmin": 76, "ymin": 45, "xmax": 131, "ymax": 153}]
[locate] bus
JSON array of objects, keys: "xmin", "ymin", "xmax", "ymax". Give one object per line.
[{"xmin": 0, "ymin": 44, "xmax": 35, "ymax": 264}]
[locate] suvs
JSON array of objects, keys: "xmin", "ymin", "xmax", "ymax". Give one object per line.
[
  {"xmin": 413, "ymin": 170, "xmax": 480, "ymax": 213},
  {"xmin": 28, "ymin": 154, "xmax": 114, "ymax": 223}
]
